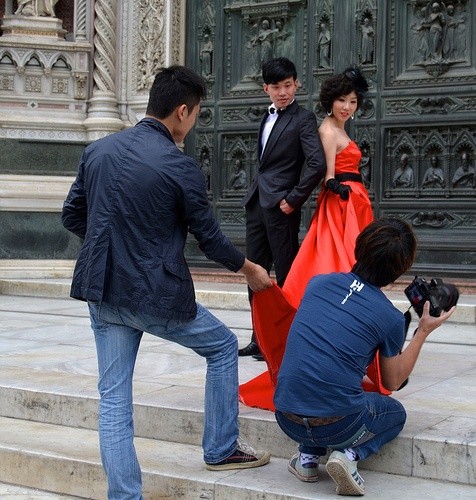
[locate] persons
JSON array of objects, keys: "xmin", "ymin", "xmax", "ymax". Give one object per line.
[
  {"xmin": 239, "ymin": 57, "xmax": 392, "ymax": 413},
  {"xmin": 273, "ymin": 213, "xmax": 457, "ymax": 496},
  {"xmin": 59, "ymin": 64, "xmax": 272, "ymax": 500}
]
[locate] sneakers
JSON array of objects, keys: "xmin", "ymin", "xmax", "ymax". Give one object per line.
[
  {"xmin": 206, "ymin": 436, "xmax": 270, "ymax": 470},
  {"xmin": 326, "ymin": 448, "xmax": 365, "ymax": 495},
  {"xmin": 288, "ymin": 452, "xmax": 319, "ymax": 481}
]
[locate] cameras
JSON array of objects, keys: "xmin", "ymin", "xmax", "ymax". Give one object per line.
[{"xmin": 404, "ymin": 276, "xmax": 460, "ymax": 318}]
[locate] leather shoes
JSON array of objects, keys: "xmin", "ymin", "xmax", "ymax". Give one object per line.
[
  {"xmin": 252, "ymin": 352, "xmax": 264, "ymax": 359},
  {"xmin": 238, "ymin": 342, "xmax": 259, "ymax": 356}
]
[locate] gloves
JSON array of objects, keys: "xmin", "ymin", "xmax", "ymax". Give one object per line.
[{"xmin": 326, "ymin": 179, "xmax": 352, "ymax": 201}]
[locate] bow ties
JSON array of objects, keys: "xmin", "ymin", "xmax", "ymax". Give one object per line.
[{"xmin": 270, "ymin": 108, "xmax": 281, "ymax": 115}]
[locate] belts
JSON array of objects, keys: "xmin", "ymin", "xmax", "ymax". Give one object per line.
[{"xmin": 281, "ymin": 412, "xmax": 346, "ymax": 426}]
